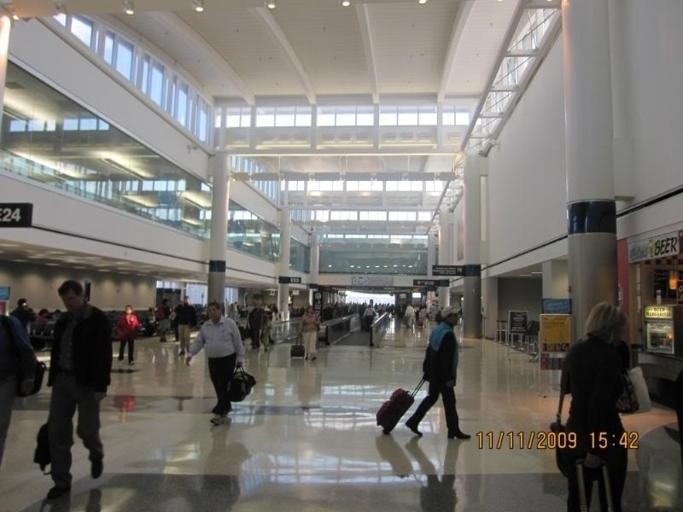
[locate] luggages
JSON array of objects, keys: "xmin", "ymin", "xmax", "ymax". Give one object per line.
[
  {"xmin": 290, "ymin": 333, "xmax": 305, "ymax": 358},
  {"xmin": 375, "ymin": 433, "xmax": 413, "ymax": 481},
  {"xmin": 375, "ymin": 388, "xmax": 415, "ymax": 435}
]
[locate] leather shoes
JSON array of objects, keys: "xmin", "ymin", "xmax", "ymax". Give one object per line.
[
  {"xmin": 447, "ymin": 430, "xmax": 471, "ymax": 440},
  {"xmin": 404, "ymin": 436, "xmax": 422, "ymax": 448},
  {"xmin": 406, "ymin": 421, "xmax": 423, "ymax": 435}
]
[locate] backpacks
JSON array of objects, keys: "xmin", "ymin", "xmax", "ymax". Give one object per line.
[{"xmin": 0, "ymin": 315, "xmax": 48, "ymax": 398}]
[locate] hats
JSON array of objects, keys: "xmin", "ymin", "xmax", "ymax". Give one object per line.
[{"xmin": 440, "ymin": 307, "xmax": 462, "ymax": 319}]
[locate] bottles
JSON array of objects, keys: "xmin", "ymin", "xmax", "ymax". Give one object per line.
[
  {"xmin": 652, "ymin": 335, "xmax": 672, "ymax": 347},
  {"xmin": 656, "ymin": 290, "xmax": 662, "ymax": 306}
]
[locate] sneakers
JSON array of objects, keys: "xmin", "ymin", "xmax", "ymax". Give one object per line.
[
  {"xmin": 46, "ymin": 453, "xmax": 104, "ymax": 501},
  {"xmin": 210, "ymin": 414, "xmax": 224, "ymax": 426}
]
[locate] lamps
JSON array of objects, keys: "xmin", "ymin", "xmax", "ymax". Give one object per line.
[
  {"xmin": 55, "ymin": 2, "xmax": 68, "ymax": 19},
  {"xmin": 122, "ymin": 0, "xmax": 135, "ymax": 17},
  {"xmin": 192, "ymin": 0, "xmax": 205, "ymax": 12},
  {"xmin": 264, "ymin": 0, "xmax": 277, "ymax": 10}
]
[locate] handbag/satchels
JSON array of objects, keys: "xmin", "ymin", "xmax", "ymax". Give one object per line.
[
  {"xmin": 615, "ymin": 365, "xmax": 652, "ymax": 415},
  {"xmin": 33, "ymin": 424, "xmax": 50, "ymax": 473},
  {"xmin": 224, "ymin": 366, "xmax": 257, "ymax": 403}
]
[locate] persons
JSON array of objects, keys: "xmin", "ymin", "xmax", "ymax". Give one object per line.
[
  {"xmin": 1, "ymin": 314, "xmax": 40, "ymax": 467},
  {"xmin": 186, "ymin": 301, "xmax": 245, "ymax": 426},
  {"xmin": 405, "ymin": 304, "xmax": 473, "ymax": 440},
  {"xmin": 552, "ymin": 301, "xmax": 653, "ymax": 512},
  {"xmin": 47, "ymin": 280, "xmax": 115, "ymax": 500},
  {"xmin": 29, "ymin": 298, "xmax": 466, "ymax": 366},
  {"xmin": 11, "ymin": 298, "xmax": 38, "ymax": 331}
]
